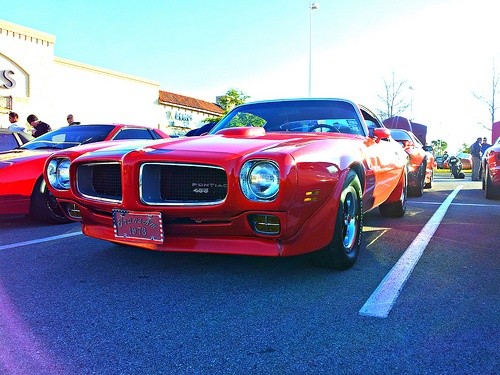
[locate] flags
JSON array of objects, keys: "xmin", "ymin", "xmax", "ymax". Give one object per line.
[{"xmin": 312, "ymin": 2, "xmax": 321, "ymax": 10}]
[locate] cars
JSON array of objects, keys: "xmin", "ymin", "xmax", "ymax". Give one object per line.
[
  {"xmin": 434, "ymin": 153, "xmax": 473, "ymax": 170},
  {"xmin": 0, "ymin": 124, "xmax": 171, "ymax": 225},
  {"xmin": 482, "ymin": 136, "xmax": 500, "ymax": 199},
  {"xmin": 50, "ymin": 97, "xmax": 435, "ymax": 271}
]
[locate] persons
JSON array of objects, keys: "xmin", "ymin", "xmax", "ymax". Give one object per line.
[
  {"xmin": 67, "ymin": 113, "xmax": 81, "ymax": 127},
  {"xmin": 479, "ymin": 137, "xmax": 490, "ymax": 179},
  {"xmin": 443, "ymin": 151, "xmax": 449, "ymax": 169},
  {"xmin": 470, "ymin": 137, "xmax": 482, "ymax": 182},
  {"xmin": 8, "ymin": 113, "xmax": 25, "ymax": 132},
  {"xmin": 27, "ymin": 114, "xmax": 53, "ymax": 138}
]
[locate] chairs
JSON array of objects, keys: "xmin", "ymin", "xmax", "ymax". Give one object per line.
[{"xmin": 329, "ymin": 125, "xmax": 352, "ymax": 134}]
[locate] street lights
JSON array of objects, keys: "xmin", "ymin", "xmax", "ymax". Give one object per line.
[{"xmin": 308, "ymin": 0, "xmax": 320, "ymax": 99}]
